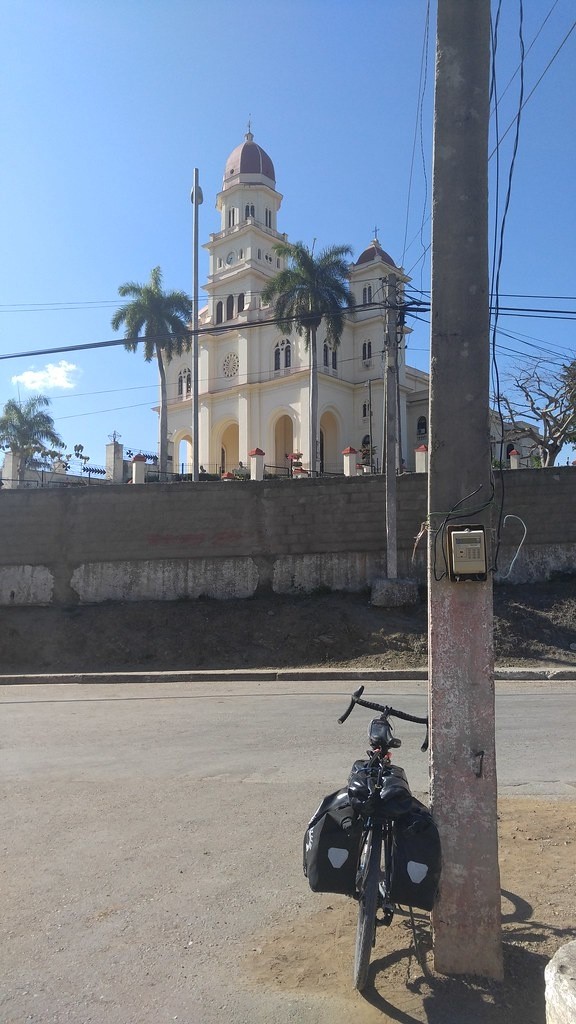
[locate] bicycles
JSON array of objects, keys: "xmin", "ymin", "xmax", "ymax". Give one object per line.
[{"xmin": 336, "ymin": 685, "xmax": 428, "ymax": 990}]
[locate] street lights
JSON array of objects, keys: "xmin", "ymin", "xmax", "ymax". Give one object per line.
[
  {"xmin": 191, "ymin": 170, "xmax": 203, "ymax": 481},
  {"xmin": 365, "ymin": 378, "xmax": 374, "ymax": 475}
]
